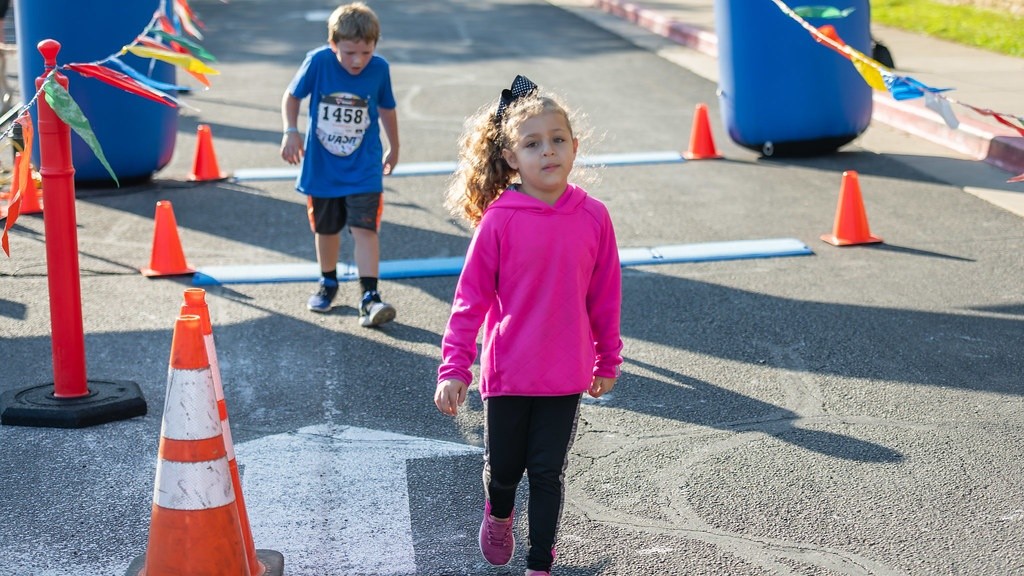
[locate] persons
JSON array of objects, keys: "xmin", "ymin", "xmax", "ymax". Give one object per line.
[
  {"xmin": 280, "ymin": 4, "xmax": 400, "ymax": 327},
  {"xmin": 432, "ymin": 74, "xmax": 624, "ymax": 576}
]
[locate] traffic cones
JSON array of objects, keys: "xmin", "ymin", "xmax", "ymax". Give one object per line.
[
  {"xmin": 121, "ymin": 314, "xmax": 255, "ymax": 576},
  {"xmin": 820, "ymin": 169, "xmax": 884, "ymax": 248},
  {"xmin": 681, "ymin": 102, "xmax": 725, "ymax": 160},
  {"xmin": 140, "ymin": 199, "xmax": 197, "ymax": 276},
  {"xmin": 184, "ymin": 122, "xmax": 230, "ymax": 185},
  {"xmin": 174, "ymin": 287, "xmax": 287, "ymax": 575}
]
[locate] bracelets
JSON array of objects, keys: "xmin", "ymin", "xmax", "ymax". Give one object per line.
[{"xmin": 282, "ymin": 127, "xmax": 299, "ymax": 134}]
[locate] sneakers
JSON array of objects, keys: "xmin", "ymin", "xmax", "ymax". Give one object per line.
[
  {"xmin": 525, "ymin": 548, "xmax": 556, "ymax": 576},
  {"xmin": 307, "ymin": 278, "xmax": 338, "ymax": 312},
  {"xmin": 479, "ymin": 498, "xmax": 515, "ymax": 566},
  {"xmin": 358, "ymin": 291, "xmax": 395, "ymax": 327}
]
